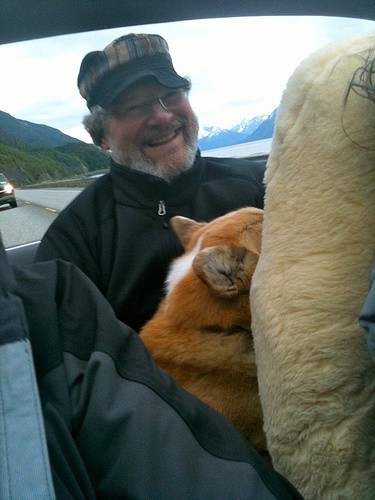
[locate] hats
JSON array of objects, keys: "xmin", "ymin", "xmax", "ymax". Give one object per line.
[{"xmin": 79, "ymin": 35, "xmax": 189, "ymax": 107}]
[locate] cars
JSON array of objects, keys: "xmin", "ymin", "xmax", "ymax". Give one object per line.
[{"xmin": 0, "ymin": 173, "xmax": 18, "ymax": 208}]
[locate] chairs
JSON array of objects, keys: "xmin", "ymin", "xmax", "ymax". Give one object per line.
[{"xmin": 249, "ymin": 32, "xmax": 375, "ymax": 500}]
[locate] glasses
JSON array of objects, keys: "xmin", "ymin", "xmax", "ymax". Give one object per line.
[{"xmin": 97, "ymin": 90, "xmax": 191, "ymax": 116}]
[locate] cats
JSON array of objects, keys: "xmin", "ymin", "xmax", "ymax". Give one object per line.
[{"xmin": 138, "ymin": 207, "xmax": 272, "ymax": 463}]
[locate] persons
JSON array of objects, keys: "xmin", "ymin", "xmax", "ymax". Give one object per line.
[
  {"xmin": 0, "ymin": 225, "xmax": 307, "ymax": 500},
  {"xmin": 33, "ymin": 33, "xmax": 268, "ymax": 333}
]
